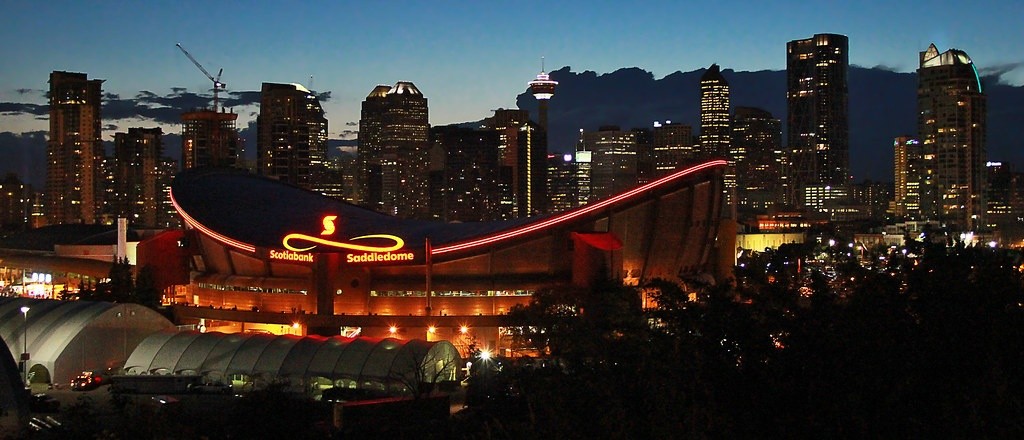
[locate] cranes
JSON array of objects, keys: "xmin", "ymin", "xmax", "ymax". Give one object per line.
[{"xmin": 176, "ymin": 43, "xmax": 226, "ymax": 111}]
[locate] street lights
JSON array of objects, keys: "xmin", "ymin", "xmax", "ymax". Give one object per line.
[{"xmin": 20, "ymin": 305, "xmax": 31, "ymax": 388}]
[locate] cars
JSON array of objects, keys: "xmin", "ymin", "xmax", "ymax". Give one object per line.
[
  {"xmin": 70, "ymin": 377, "xmax": 98, "ymax": 391},
  {"xmin": 193, "ymin": 380, "xmax": 231, "ymax": 395},
  {"xmin": 31, "ymin": 393, "xmax": 61, "ymax": 412}
]
